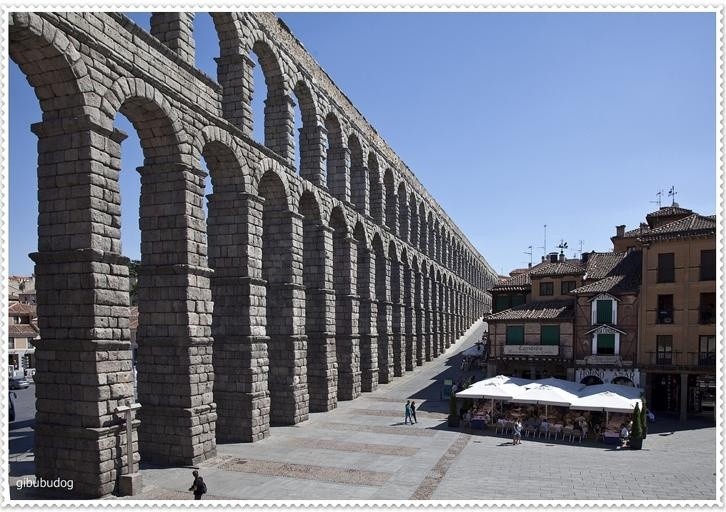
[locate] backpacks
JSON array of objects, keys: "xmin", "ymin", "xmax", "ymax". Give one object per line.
[{"xmin": 196, "ymin": 477, "xmax": 207, "ymax": 494}]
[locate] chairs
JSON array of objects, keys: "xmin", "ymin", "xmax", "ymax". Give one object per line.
[{"xmin": 494, "ymin": 422, "xmax": 583, "ymax": 445}]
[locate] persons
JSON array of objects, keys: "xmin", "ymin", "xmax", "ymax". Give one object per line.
[
  {"xmin": 188, "ymin": 471, "xmax": 207, "ymax": 500},
  {"xmin": 513, "ymin": 418, "xmax": 523, "ymax": 445},
  {"xmin": 592, "ymin": 424, "xmax": 601, "ymax": 440},
  {"xmin": 405, "ymin": 400, "xmax": 418, "ymax": 424},
  {"xmin": 615, "ymin": 423, "xmax": 629, "ymax": 450}
]
